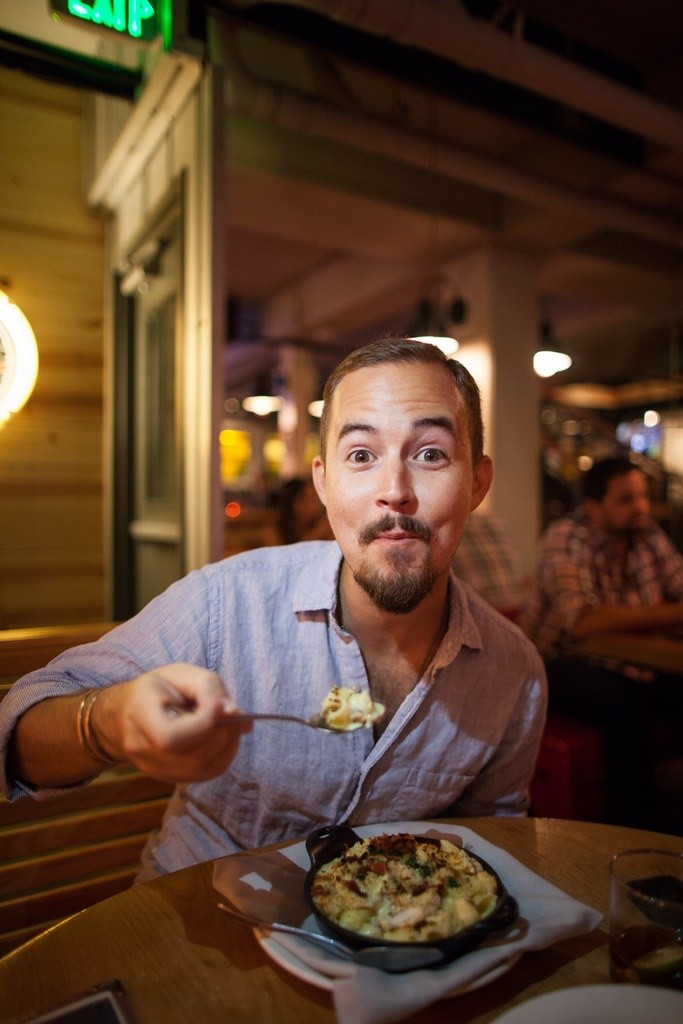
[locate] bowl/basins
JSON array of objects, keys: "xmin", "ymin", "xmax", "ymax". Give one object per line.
[{"xmin": 303, "ymin": 823, "xmax": 519, "ymax": 974}]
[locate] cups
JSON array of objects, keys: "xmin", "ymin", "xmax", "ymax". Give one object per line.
[{"xmin": 607, "ymin": 848, "xmax": 683, "ymax": 989}]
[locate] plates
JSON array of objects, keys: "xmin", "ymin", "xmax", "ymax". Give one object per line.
[
  {"xmin": 489, "ymin": 984, "xmax": 683, "ymax": 1024},
  {"xmin": 249, "ymin": 917, "xmax": 522, "ymax": 996}
]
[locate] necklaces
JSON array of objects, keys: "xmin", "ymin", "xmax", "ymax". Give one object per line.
[{"xmin": 338, "ymin": 583, "xmax": 450, "ymax": 691}]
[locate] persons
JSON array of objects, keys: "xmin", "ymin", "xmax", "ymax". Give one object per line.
[
  {"xmin": 0, "ymin": 337, "xmax": 547, "ymax": 888},
  {"xmin": 275, "ymin": 456, "xmax": 683, "ymax": 838}
]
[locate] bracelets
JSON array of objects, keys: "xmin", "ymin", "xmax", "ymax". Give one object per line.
[{"xmin": 78, "ymin": 689, "xmax": 123, "ymax": 765}]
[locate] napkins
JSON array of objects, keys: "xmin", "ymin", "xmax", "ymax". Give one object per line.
[{"xmin": 213, "ymin": 821, "xmax": 605, "ymax": 1023}]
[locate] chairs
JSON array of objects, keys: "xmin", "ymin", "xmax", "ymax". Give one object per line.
[
  {"xmin": 0, "ymin": 611, "xmax": 187, "ymax": 959},
  {"xmin": 492, "ymin": 603, "xmax": 610, "ymax": 817}
]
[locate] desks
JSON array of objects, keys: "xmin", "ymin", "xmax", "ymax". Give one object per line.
[
  {"xmin": 597, "ymin": 613, "xmax": 683, "ymax": 789},
  {"xmin": 1, "ymin": 816, "xmax": 682, "ymax": 1024}
]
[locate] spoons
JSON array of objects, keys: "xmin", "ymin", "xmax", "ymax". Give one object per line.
[
  {"xmin": 217, "ymin": 903, "xmax": 444, "ymax": 971},
  {"xmin": 165, "ymin": 701, "xmax": 386, "ymax": 733}
]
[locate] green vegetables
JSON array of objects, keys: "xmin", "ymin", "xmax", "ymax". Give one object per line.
[{"xmin": 391, "ymin": 849, "xmax": 461, "ymax": 888}]
